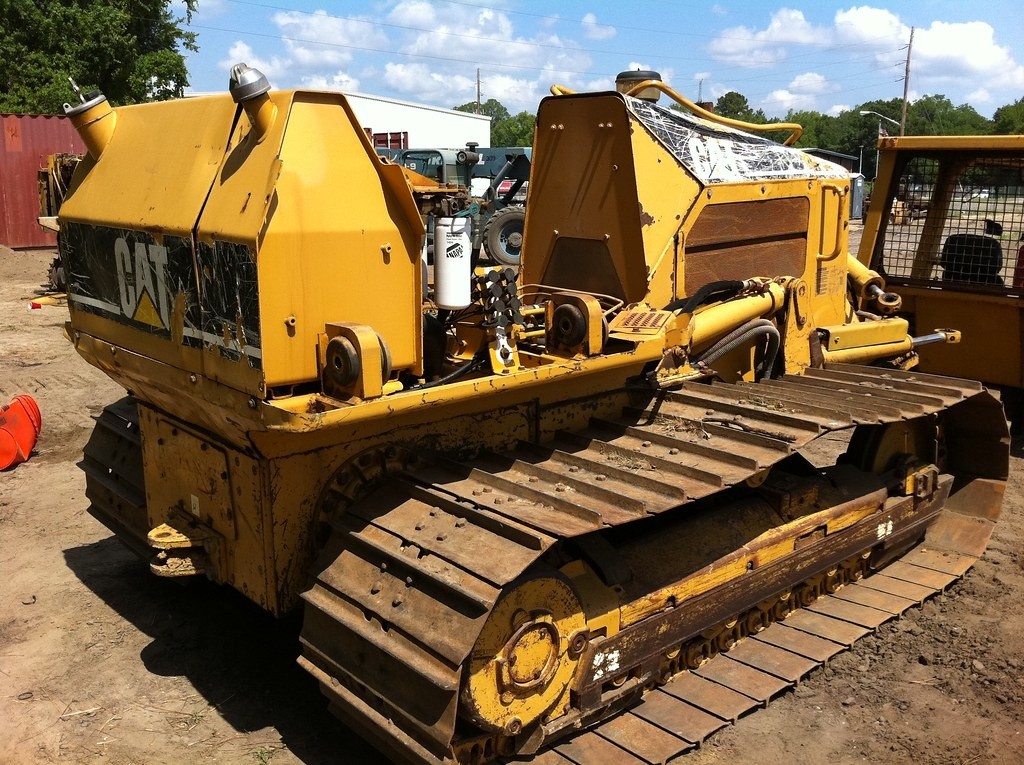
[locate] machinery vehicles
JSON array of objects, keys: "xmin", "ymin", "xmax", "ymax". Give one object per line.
[
  {"xmin": 862, "ymin": 175, "xmax": 930, "ymax": 226},
  {"xmin": 36, "ymin": 63, "xmax": 1024, "ymax": 765},
  {"xmin": 29, "ymin": 142, "xmax": 534, "ymax": 305}
]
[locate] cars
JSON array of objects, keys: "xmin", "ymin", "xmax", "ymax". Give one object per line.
[{"xmin": 971, "ymin": 190, "xmax": 989, "ymax": 199}]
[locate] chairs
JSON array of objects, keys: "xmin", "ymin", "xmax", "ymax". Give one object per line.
[{"xmin": 943, "ymin": 233, "xmax": 1005, "ymax": 285}]
